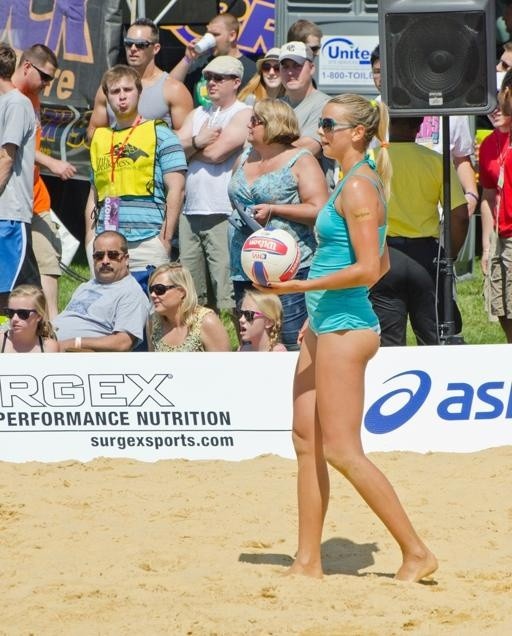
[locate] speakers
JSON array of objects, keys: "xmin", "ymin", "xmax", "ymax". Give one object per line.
[{"xmin": 376, "ymin": 1, "xmax": 499, "ymax": 114}]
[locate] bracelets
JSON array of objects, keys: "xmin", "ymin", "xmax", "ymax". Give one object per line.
[{"xmin": 75, "ymin": 336, "xmax": 81, "ymax": 350}]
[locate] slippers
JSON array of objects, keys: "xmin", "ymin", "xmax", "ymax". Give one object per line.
[
  {"xmin": 233, "ymin": 199, "xmax": 263, "ymax": 232},
  {"xmin": 228, "ymin": 207, "xmax": 250, "ymax": 233}
]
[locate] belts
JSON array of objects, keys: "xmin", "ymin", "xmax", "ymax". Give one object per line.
[{"xmin": 387, "ymin": 236, "xmax": 429, "ymax": 245}]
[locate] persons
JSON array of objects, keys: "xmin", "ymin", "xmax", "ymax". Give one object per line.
[
  {"xmin": 86, "ymin": 11, "xmax": 335, "ymax": 353},
  {"xmin": 252, "ymin": 94, "xmax": 438, "ymax": 585},
  {"xmin": 51, "ymin": 228, "xmax": 152, "ymax": 353},
  {"xmin": 371, "ymin": 39, "xmax": 511, "ymax": 343},
  {"xmin": 0, "ymin": 39, "xmax": 76, "ymax": 325},
  {"xmin": 0, "ymin": 283, "xmax": 60, "ymax": 353}
]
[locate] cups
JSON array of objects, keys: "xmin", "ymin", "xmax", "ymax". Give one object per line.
[
  {"xmin": 210, "ymin": 104, "xmax": 226, "ymax": 129},
  {"xmin": 193, "ymin": 31, "xmax": 217, "ymax": 54}
]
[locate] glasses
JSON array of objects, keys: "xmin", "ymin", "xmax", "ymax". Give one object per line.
[
  {"xmin": 262, "ymin": 62, "xmax": 279, "ymax": 70},
  {"xmin": 4, "ymin": 308, "xmax": 37, "ymax": 319},
  {"xmin": 149, "ymin": 284, "xmax": 176, "ymax": 296},
  {"xmin": 235, "ymin": 309, "xmax": 263, "ymax": 320},
  {"xmin": 318, "ymin": 116, "xmax": 356, "ymax": 132},
  {"xmin": 93, "ymin": 250, "xmax": 126, "ymax": 260},
  {"xmin": 205, "ymin": 73, "xmax": 224, "ymax": 80},
  {"xmin": 26, "ymin": 60, "xmax": 54, "ymax": 82},
  {"xmin": 123, "ymin": 37, "xmax": 155, "ymax": 49}
]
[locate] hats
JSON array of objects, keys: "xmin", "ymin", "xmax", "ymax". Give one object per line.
[
  {"xmin": 202, "ymin": 54, "xmax": 244, "ymax": 79},
  {"xmin": 256, "ymin": 47, "xmax": 280, "ymax": 72},
  {"xmin": 311, "ymin": 45, "xmax": 320, "ymax": 50},
  {"xmin": 280, "ymin": 40, "xmax": 314, "ymax": 65}
]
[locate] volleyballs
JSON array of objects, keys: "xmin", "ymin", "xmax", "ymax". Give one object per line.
[{"xmin": 240, "ymin": 226, "xmax": 301, "ymax": 286}]
